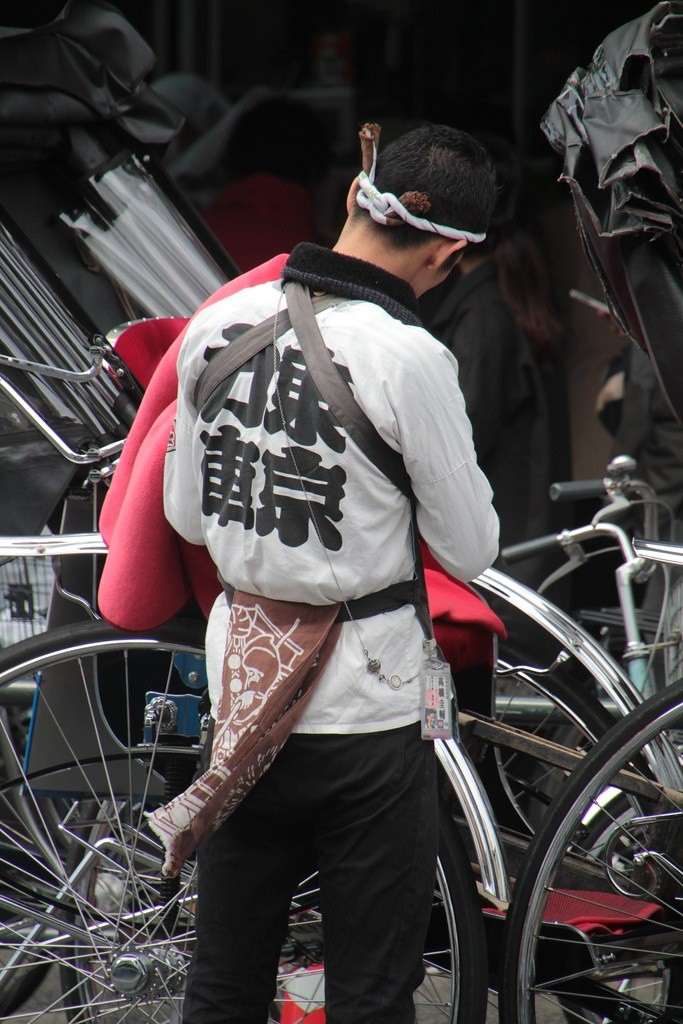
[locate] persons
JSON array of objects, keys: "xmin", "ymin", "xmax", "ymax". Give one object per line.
[
  {"xmin": 159, "ymin": 122, "xmax": 499, "ymax": 1024},
  {"xmin": 204, "ymin": 97, "xmax": 683, "ymax": 617}
]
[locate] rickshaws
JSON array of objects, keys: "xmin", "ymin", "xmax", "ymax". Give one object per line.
[{"xmin": 1, "ymin": 0, "xmax": 683, "ymax": 1024}]
